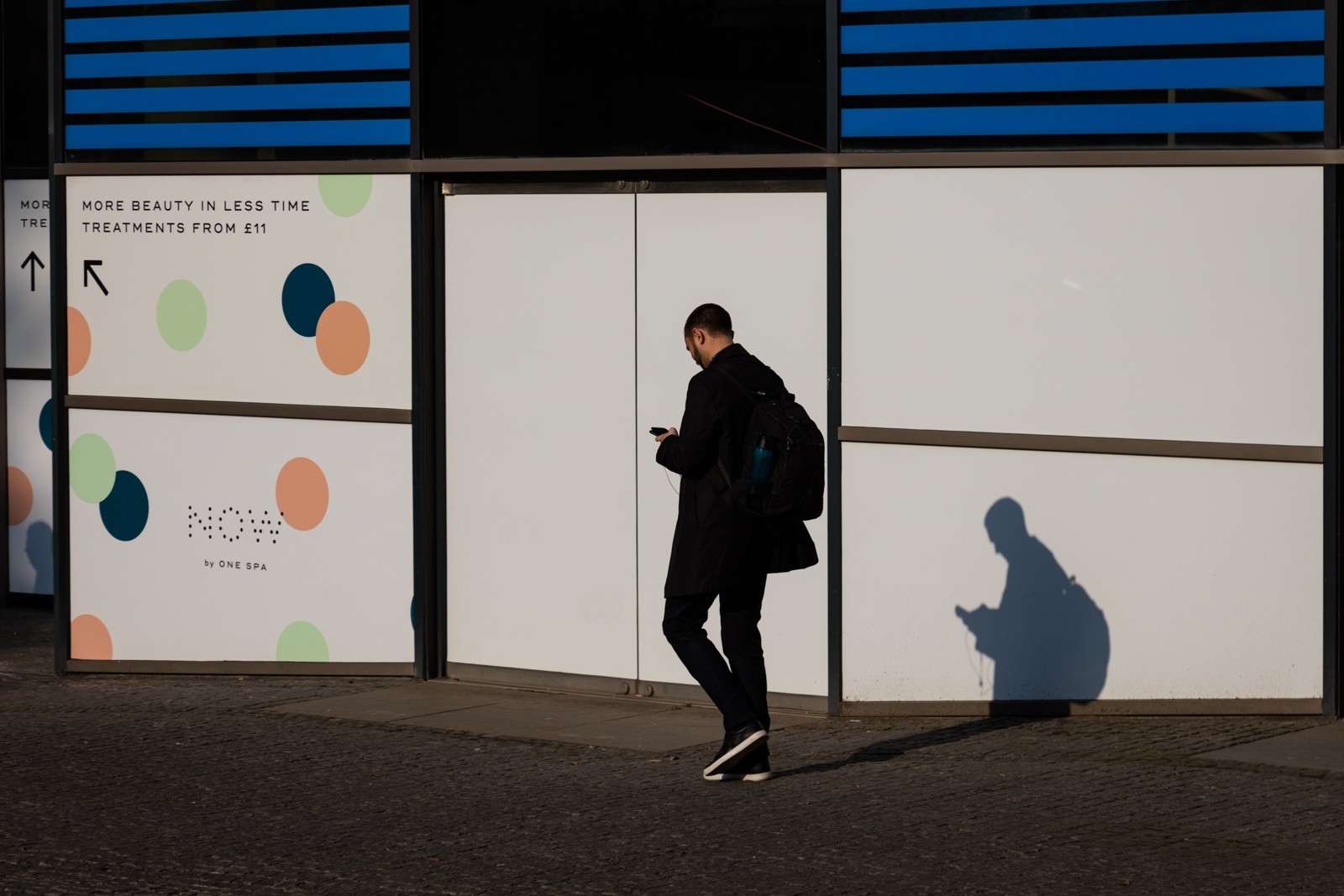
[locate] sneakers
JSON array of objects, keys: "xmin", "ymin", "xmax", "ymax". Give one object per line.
[
  {"xmin": 703, "ymin": 721, "xmax": 770, "ymax": 776},
  {"xmin": 702, "ymin": 756, "xmax": 772, "ymax": 783}
]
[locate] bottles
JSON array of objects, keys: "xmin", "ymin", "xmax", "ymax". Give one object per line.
[{"xmin": 749, "ymin": 438, "xmax": 770, "ymax": 484}]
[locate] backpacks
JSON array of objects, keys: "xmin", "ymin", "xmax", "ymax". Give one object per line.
[{"xmin": 706, "ymin": 358, "xmax": 825, "ymax": 520}]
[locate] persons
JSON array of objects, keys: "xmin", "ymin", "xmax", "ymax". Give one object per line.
[{"xmin": 656, "ymin": 303, "xmax": 819, "ymax": 781}]
